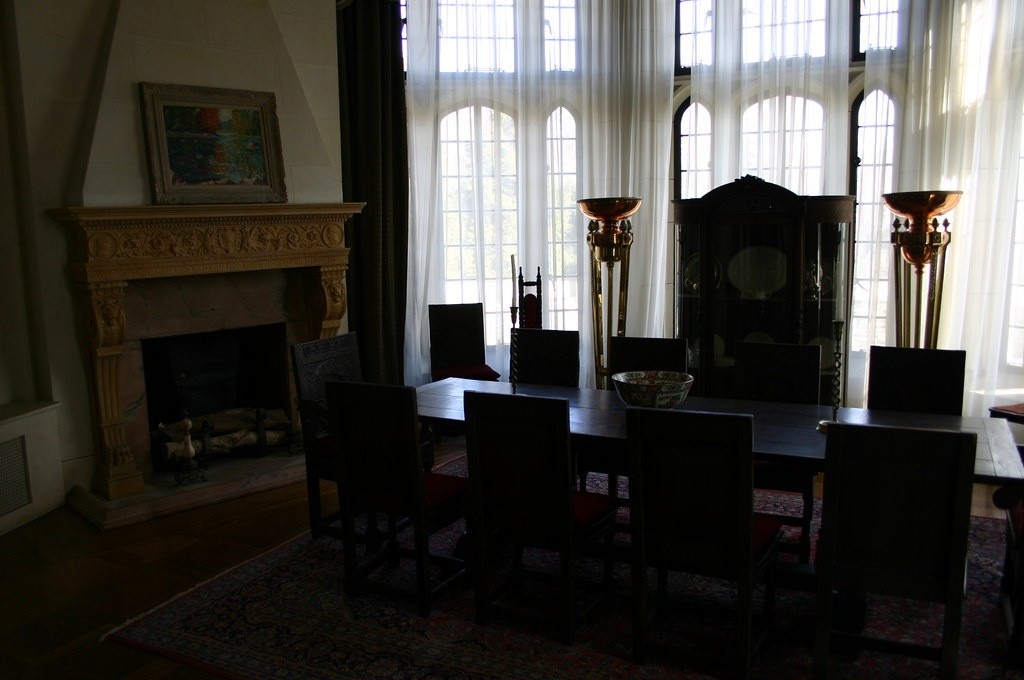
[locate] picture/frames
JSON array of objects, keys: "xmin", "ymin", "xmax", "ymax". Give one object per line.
[{"xmin": 138, "ymin": 80, "xmax": 288, "ymax": 207}]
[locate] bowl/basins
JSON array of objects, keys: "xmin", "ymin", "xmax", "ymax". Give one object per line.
[{"xmin": 611, "ymin": 370, "xmax": 694, "ymax": 409}]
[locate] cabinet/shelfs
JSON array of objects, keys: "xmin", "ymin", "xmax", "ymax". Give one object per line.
[{"xmin": 671, "ymin": 175, "xmax": 857, "ymax": 410}]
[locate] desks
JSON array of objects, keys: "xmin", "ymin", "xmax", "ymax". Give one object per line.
[{"xmin": 414, "ymin": 377, "xmax": 1024, "ymax": 661}]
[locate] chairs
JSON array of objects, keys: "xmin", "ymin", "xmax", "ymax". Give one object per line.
[
  {"xmin": 101, "ymin": 450, "xmax": 1024, "ymax": 680},
  {"xmin": 290, "ymin": 267, "xmax": 979, "ymax": 679}
]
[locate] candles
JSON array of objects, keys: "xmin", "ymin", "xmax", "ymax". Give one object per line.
[
  {"xmin": 835, "ymin": 260, "xmax": 844, "ymax": 322},
  {"xmin": 511, "ymin": 255, "xmax": 516, "ymax": 307}
]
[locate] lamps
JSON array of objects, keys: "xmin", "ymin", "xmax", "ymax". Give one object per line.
[
  {"xmin": 882, "ymin": 191, "xmax": 952, "ymax": 351},
  {"xmin": 578, "ymin": 197, "xmax": 640, "ymax": 390}
]
